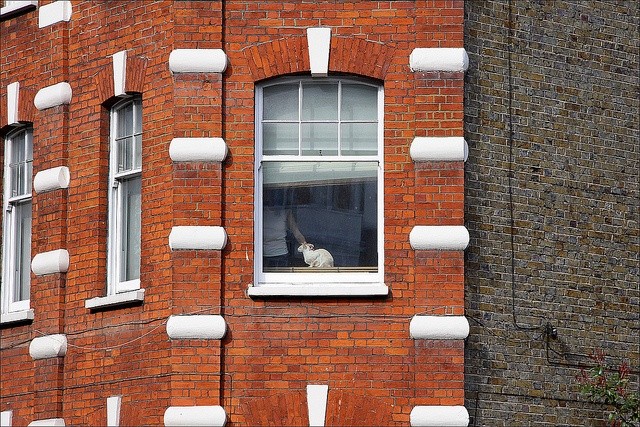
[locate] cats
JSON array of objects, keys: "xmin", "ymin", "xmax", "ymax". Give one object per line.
[{"xmin": 297, "ymin": 242, "xmax": 334, "ymax": 269}]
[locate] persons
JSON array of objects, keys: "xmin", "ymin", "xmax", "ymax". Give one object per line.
[{"xmin": 263, "ymin": 188, "xmax": 314, "ymax": 267}]
